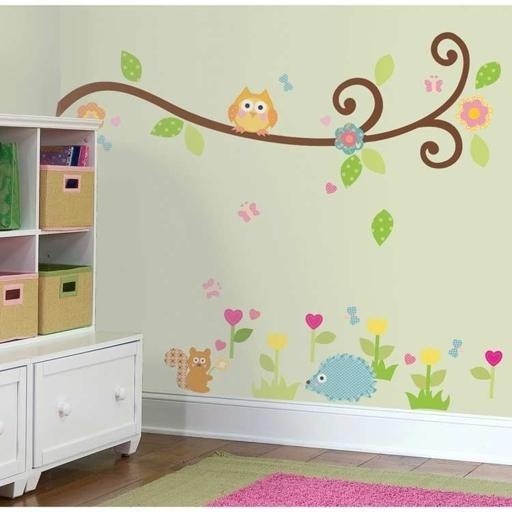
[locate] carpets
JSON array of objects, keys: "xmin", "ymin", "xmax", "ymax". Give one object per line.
[
  {"xmin": 99, "ymin": 452, "xmax": 512, "ymax": 507},
  {"xmin": 203, "ymin": 472, "xmax": 512, "ymax": 508}
]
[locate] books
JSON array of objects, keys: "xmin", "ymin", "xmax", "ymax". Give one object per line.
[{"xmin": 40, "ymin": 144, "xmax": 89, "ymax": 166}]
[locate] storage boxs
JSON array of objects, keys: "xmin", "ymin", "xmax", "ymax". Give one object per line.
[
  {"xmin": 37, "ymin": 261, "xmax": 94, "ymax": 335},
  {"xmin": 39, "ymin": 164, "xmax": 96, "ymax": 232},
  {"xmin": 0, "ymin": 270, "xmax": 40, "ymax": 345}
]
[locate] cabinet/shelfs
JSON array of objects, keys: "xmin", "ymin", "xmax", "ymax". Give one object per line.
[
  {"xmin": 0, "ymin": 113, "xmax": 103, "ymax": 351},
  {"xmin": 0, "ymin": 330, "xmax": 145, "ymax": 500}
]
